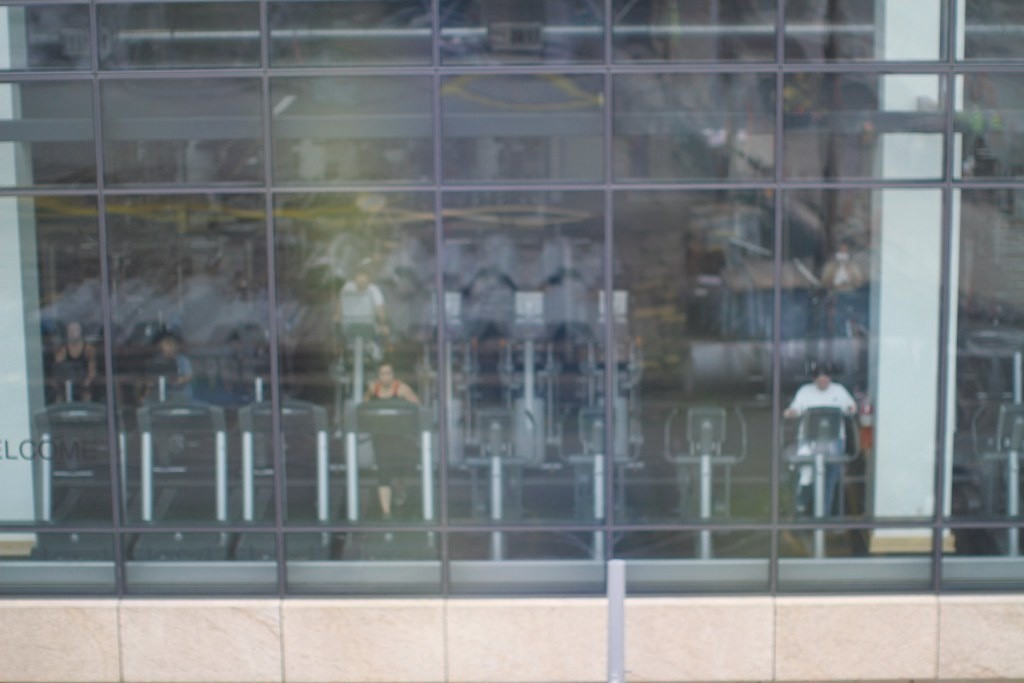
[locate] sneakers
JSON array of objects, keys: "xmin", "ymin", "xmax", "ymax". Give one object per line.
[
  {"xmin": 393, "ymin": 487, "xmax": 405, "ymax": 508},
  {"xmin": 382, "ymin": 517, "xmax": 397, "ymax": 542}
]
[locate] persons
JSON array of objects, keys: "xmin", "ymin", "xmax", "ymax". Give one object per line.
[
  {"xmin": 784, "ymin": 365, "xmax": 857, "ymax": 528},
  {"xmin": 143, "ymin": 332, "xmax": 202, "ymax": 405},
  {"xmin": 332, "ymin": 271, "xmax": 391, "ymax": 369},
  {"xmin": 366, "ymin": 364, "xmax": 423, "ymax": 523},
  {"xmin": 820, "ymin": 241, "xmax": 863, "ymax": 337},
  {"xmin": 217, "ymin": 332, "xmax": 256, "ymax": 401},
  {"xmin": 54, "ymin": 320, "xmax": 99, "ymax": 402}
]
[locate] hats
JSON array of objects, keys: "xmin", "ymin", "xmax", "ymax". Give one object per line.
[{"xmin": 151, "ymin": 324, "xmax": 183, "ymax": 343}]
